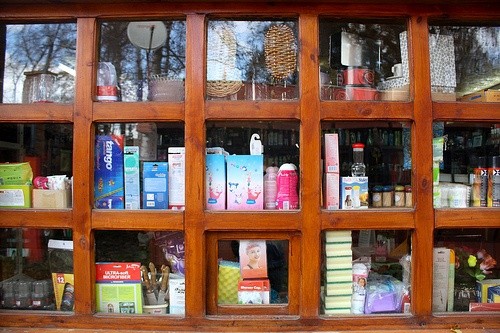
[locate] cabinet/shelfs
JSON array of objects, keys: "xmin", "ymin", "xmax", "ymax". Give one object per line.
[{"xmin": 0, "ymin": 0, "xmax": 500, "ymax": 333}]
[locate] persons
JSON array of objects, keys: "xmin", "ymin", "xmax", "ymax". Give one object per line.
[{"xmin": 244, "ymin": 240, "xmax": 264, "ymax": 269}]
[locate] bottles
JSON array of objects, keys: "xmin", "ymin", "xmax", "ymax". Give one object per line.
[
  {"xmin": 96, "ymin": 61, "xmax": 119, "ymax": 102},
  {"xmin": 350, "ymin": 143, "xmax": 366, "ymax": 177},
  {"xmin": 263, "ymin": 163, "xmax": 297, "ymax": 210}
]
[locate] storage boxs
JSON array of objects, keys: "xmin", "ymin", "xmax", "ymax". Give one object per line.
[
  {"xmin": 205, "ymin": 154, "xmax": 226, "ymax": 211},
  {"xmin": 47, "ymin": 239, "xmax": 75, "ymax": 311},
  {"xmin": 93, "ymin": 134, "xmax": 124, "ymax": 209},
  {"xmin": 124, "ymin": 146, "xmax": 140, "ymax": 209},
  {"xmin": 0, "ymin": 184, "xmax": 34, "ymax": 209},
  {"xmin": 226, "ymin": 154, "xmax": 263, "ymax": 211},
  {"xmin": 96, "ymin": 262, "xmax": 144, "ymax": 314},
  {"xmin": 168, "ymin": 147, "xmax": 186, "ymax": 211},
  {"xmin": 142, "ymin": 162, "xmax": 168, "ymax": 210},
  {"xmin": 32, "ymin": 185, "xmax": 72, "ymax": 209}
]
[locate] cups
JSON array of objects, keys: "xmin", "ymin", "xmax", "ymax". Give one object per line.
[{"xmin": 29, "ymin": 74, "xmax": 56, "ymax": 103}]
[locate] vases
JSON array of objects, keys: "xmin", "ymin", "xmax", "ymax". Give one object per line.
[{"xmin": 453, "ymin": 280, "xmax": 480, "ymax": 312}]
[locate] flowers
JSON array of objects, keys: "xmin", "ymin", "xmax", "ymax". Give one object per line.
[{"xmin": 438, "ymin": 239, "xmax": 496, "ymax": 283}]
